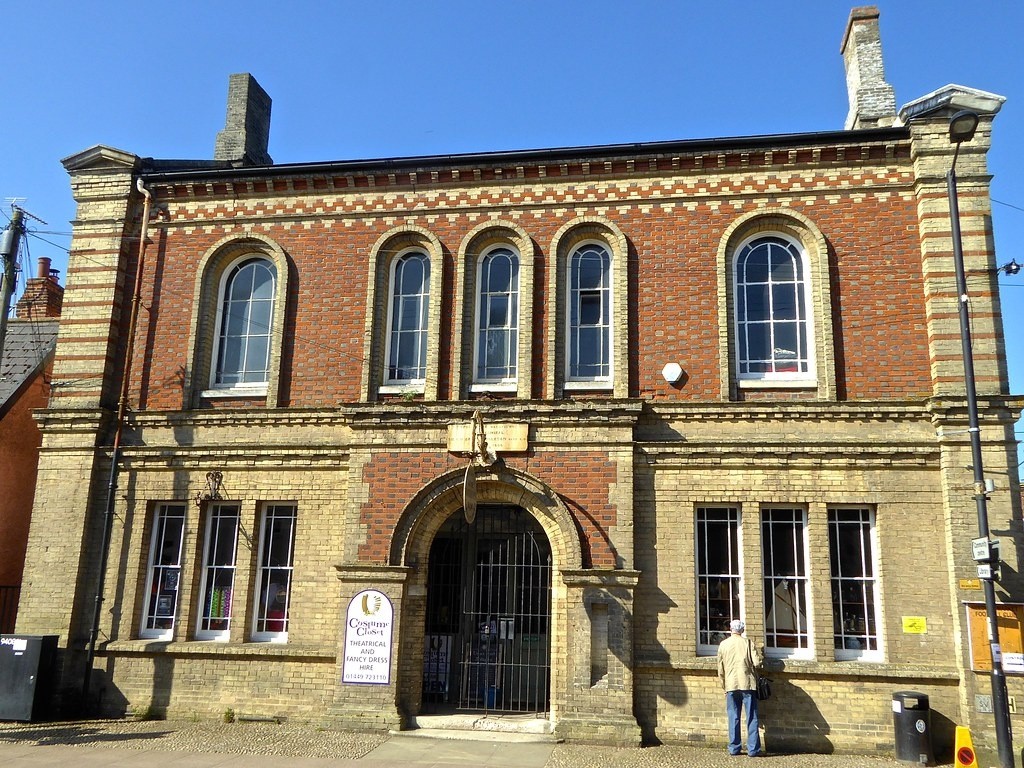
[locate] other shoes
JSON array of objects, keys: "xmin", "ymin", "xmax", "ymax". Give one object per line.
[{"xmin": 748, "ymin": 750, "xmax": 768, "ymax": 757}]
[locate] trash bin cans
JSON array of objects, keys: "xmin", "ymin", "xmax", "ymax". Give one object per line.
[
  {"xmin": 0, "ymin": 635, "xmax": 61, "ymax": 722},
  {"xmin": 892, "ymin": 690, "xmax": 936, "ymax": 763}
]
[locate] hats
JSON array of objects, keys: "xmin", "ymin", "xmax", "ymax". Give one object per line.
[{"xmin": 730, "ymin": 620, "xmax": 745, "ymax": 632}]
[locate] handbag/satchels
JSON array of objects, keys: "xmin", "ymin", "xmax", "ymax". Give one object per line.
[{"xmin": 756, "ymin": 674, "xmax": 774, "ymax": 700}]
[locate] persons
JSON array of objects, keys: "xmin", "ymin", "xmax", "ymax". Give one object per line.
[{"xmin": 717, "ymin": 619, "xmax": 764, "ymax": 757}]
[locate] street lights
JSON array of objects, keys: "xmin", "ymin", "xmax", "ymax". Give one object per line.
[{"xmin": 942, "ymin": 112, "xmax": 1017, "ymax": 768}]
